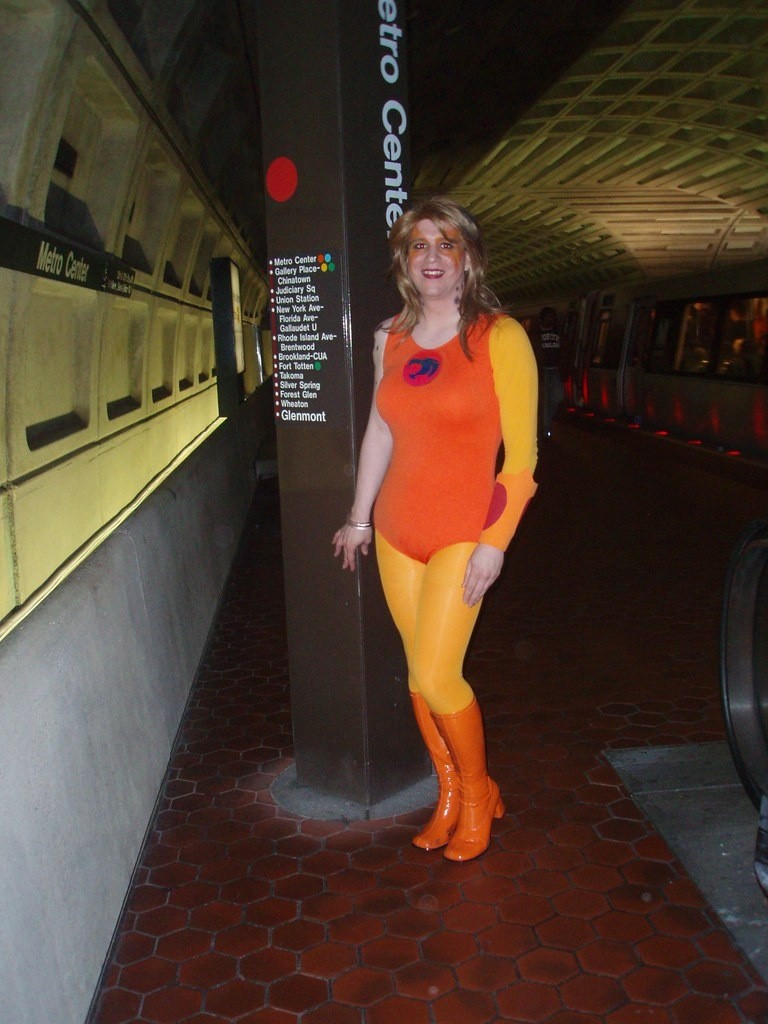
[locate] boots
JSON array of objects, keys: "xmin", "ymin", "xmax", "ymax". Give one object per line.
[
  {"xmin": 410, "ymin": 691, "xmax": 461, "ymax": 851},
  {"xmin": 429, "ymin": 695, "xmax": 506, "ymax": 862}
]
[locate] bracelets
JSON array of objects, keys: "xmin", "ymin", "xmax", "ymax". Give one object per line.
[{"xmin": 346, "ymin": 517, "xmax": 371, "ymax": 530}]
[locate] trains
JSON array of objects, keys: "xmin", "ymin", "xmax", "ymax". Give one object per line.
[{"xmin": 502, "ymin": 255, "xmax": 768, "ymax": 463}]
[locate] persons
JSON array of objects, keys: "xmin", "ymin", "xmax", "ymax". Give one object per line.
[
  {"xmin": 532, "ymin": 306, "xmax": 568, "ymax": 432},
  {"xmin": 332, "ymin": 200, "xmax": 539, "ymax": 861}
]
